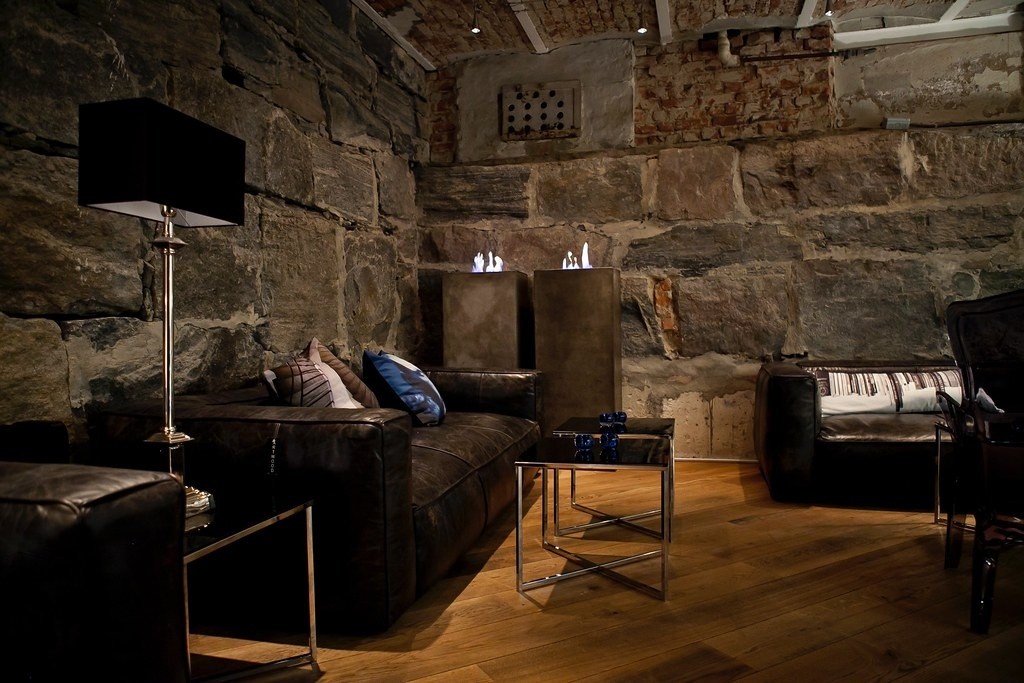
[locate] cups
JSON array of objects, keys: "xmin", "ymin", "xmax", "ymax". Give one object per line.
[
  {"xmin": 612, "ymin": 412, "xmax": 627, "ymax": 423},
  {"xmin": 599, "ymin": 413, "xmax": 613, "ymax": 423},
  {"xmin": 599, "ymin": 434, "xmax": 620, "ymax": 449},
  {"xmin": 574, "ymin": 434, "xmax": 594, "ymax": 448}
]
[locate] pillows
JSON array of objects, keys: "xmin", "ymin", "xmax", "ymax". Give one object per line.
[
  {"xmin": 362, "ymin": 350, "xmax": 447, "ymax": 427},
  {"xmin": 815, "ymin": 370, "xmax": 963, "ymax": 417},
  {"xmin": 260, "ymin": 336, "xmax": 380, "ymax": 409}
]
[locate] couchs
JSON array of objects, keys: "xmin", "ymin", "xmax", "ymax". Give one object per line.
[
  {"xmin": 753, "ymin": 360, "xmax": 958, "ymax": 504},
  {"xmin": 99, "ymin": 367, "xmax": 544, "ymax": 634},
  {"xmin": 0, "ymin": 461, "xmax": 187, "ymax": 683}
]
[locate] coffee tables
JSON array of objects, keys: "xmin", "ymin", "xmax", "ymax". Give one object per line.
[{"xmin": 514, "ymin": 417, "xmax": 675, "ymax": 602}]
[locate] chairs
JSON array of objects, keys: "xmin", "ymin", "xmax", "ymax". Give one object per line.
[{"xmin": 936, "ymin": 288, "xmax": 1024, "ymax": 633}]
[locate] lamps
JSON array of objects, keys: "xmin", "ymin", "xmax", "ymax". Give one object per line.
[{"xmin": 77, "ymin": 97, "xmax": 245, "ymax": 517}]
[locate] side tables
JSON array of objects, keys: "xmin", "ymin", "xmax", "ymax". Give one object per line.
[{"xmin": 182, "ymin": 493, "xmax": 325, "ymax": 683}]
